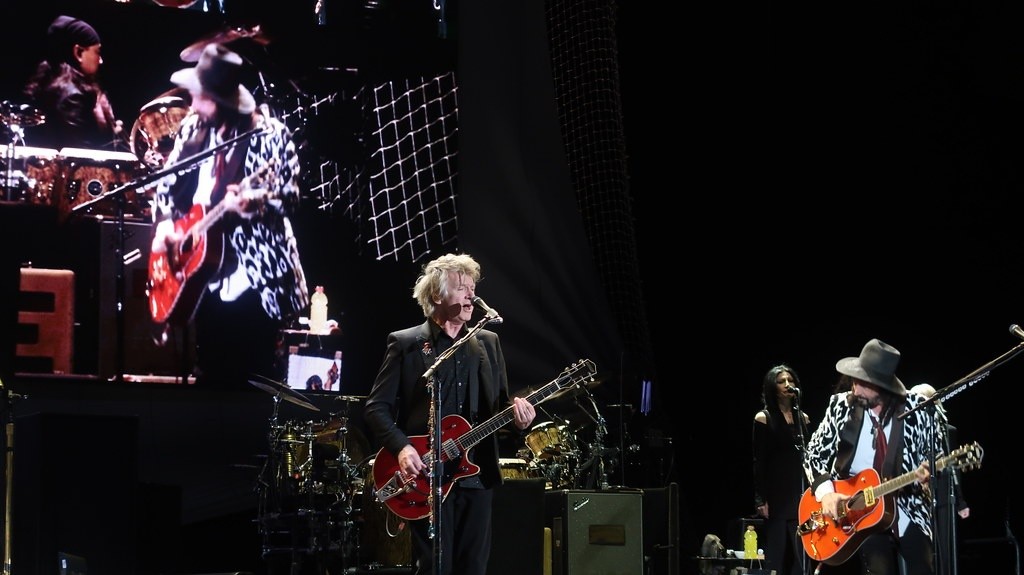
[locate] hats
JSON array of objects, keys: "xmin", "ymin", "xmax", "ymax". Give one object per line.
[
  {"xmin": 835, "ymin": 339, "xmax": 907, "ymax": 396},
  {"xmin": 50, "ymin": 16, "xmax": 100, "ymax": 55},
  {"xmin": 167, "ymin": 43, "xmax": 258, "ymax": 114}
]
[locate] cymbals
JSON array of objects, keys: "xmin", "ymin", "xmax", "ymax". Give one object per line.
[{"xmin": 247, "ymin": 374, "xmax": 322, "ymax": 412}]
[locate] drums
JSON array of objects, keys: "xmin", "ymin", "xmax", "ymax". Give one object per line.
[
  {"xmin": 499, "ymin": 414, "xmax": 578, "ymax": 488},
  {"xmin": 349, "ymin": 457, "xmax": 415, "ymax": 575},
  {"xmin": 0, "ymin": 88, "xmax": 200, "ymax": 218},
  {"xmin": 269, "ymin": 416, "xmax": 349, "ymax": 496}
]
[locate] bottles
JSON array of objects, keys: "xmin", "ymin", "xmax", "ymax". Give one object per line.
[
  {"xmin": 743, "ymin": 526, "xmax": 758, "ymax": 559},
  {"xmin": 311, "ymin": 286, "xmax": 329, "ymax": 334}
]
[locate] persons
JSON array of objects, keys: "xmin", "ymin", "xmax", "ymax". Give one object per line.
[
  {"xmin": 145, "ymin": 41, "xmax": 309, "ymax": 395},
  {"xmin": 305, "ymin": 375, "xmax": 332, "ymax": 391},
  {"xmin": 802, "ymin": 338, "xmax": 969, "ymax": 575},
  {"xmin": 367, "ymin": 253, "xmax": 535, "ymax": 575},
  {"xmin": 749, "ymin": 364, "xmax": 814, "ymax": 575},
  {"xmin": 21, "ymin": 15, "xmax": 126, "ymax": 372}
]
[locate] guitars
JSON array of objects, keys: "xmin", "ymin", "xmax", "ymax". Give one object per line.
[
  {"xmin": 797, "ymin": 440, "xmax": 984, "ymax": 566},
  {"xmin": 371, "ymin": 357, "xmax": 599, "ymax": 521},
  {"xmin": 143, "ymin": 179, "xmax": 257, "ymax": 326}
]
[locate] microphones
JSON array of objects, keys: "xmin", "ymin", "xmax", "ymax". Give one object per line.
[
  {"xmin": 259, "ymin": 103, "xmax": 273, "ymax": 134},
  {"xmin": 470, "ymin": 296, "xmax": 503, "ymax": 324},
  {"xmin": 787, "ymin": 387, "xmax": 799, "ymax": 393},
  {"xmin": 1008, "ymin": 324, "xmax": 1024, "ymax": 340}
]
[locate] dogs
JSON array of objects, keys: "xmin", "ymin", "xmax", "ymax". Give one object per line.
[{"xmin": 702, "ymin": 535, "xmax": 748, "ymax": 575}]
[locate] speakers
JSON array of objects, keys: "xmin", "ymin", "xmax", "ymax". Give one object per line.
[{"xmin": 565, "ymin": 482, "xmax": 681, "ymax": 575}]
